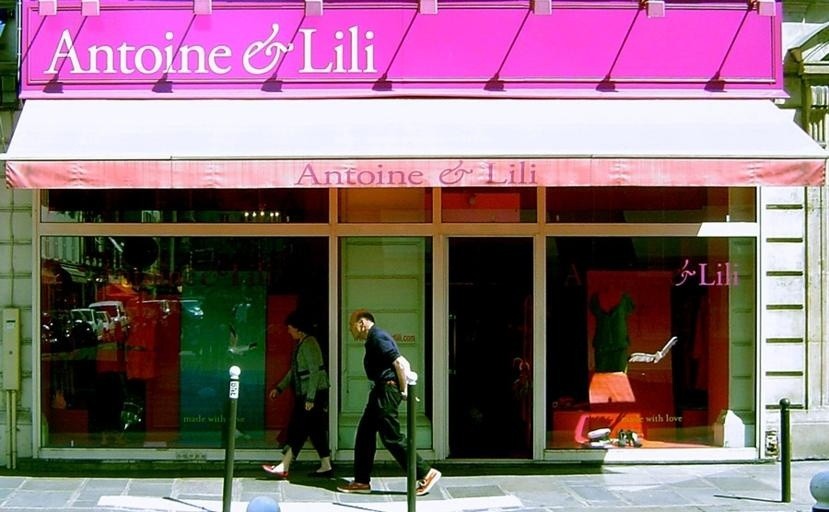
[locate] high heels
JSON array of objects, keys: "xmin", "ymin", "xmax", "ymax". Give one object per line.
[{"xmin": 628, "ymin": 430, "xmax": 642, "ymax": 448}]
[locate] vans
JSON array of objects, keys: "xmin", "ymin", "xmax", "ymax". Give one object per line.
[{"xmin": 89, "ymin": 300, "xmax": 128, "ymax": 338}]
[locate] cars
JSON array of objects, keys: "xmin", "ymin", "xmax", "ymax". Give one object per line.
[
  {"xmin": 180, "ymin": 301, "xmax": 205, "ymax": 321},
  {"xmin": 232, "ymin": 303, "xmax": 254, "ymax": 324},
  {"xmin": 42, "ymin": 307, "xmax": 114, "ymax": 352}
]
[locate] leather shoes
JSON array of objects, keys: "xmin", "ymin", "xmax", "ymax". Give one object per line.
[
  {"xmin": 618, "ymin": 428, "xmax": 627, "ymax": 446},
  {"xmin": 306, "ymin": 469, "xmax": 336, "ymax": 478},
  {"xmin": 261, "ymin": 463, "xmax": 289, "ymax": 479}
]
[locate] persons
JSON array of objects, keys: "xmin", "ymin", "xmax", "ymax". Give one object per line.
[
  {"xmin": 260, "ymin": 311, "xmax": 336, "ymax": 479},
  {"xmin": 336, "ymin": 309, "xmax": 443, "ymax": 496}
]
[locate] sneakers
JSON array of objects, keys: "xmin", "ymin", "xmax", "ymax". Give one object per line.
[
  {"xmin": 335, "ymin": 481, "xmax": 372, "ymax": 496},
  {"xmin": 414, "ymin": 467, "xmax": 442, "ymax": 496}
]
